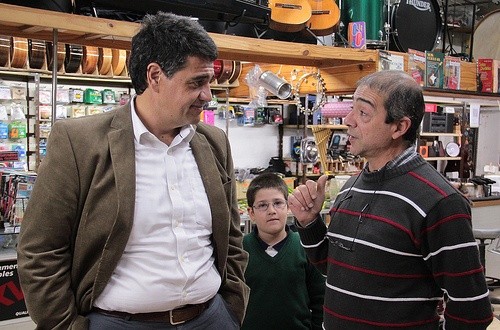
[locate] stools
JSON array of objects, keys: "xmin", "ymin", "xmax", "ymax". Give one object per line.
[{"xmin": 471, "ymin": 228, "xmax": 500, "ymax": 305}]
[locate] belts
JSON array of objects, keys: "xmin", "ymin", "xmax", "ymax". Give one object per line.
[{"xmin": 94, "ymin": 298, "xmax": 212, "ymax": 325}]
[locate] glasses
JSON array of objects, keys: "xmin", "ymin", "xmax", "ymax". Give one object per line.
[
  {"xmin": 252, "ymin": 200, "xmax": 287, "ymax": 211},
  {"xmin": 324, "ymin": 195, "xmax": 368, "ymax": 252}
]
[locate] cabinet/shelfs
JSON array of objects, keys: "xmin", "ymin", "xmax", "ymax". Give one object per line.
[{"xmin": 0, "ymin": 2, "xmax": 500, "ymax": 264}]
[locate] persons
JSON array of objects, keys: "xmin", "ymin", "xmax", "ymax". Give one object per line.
[
  {"xmin": 241, "ymin": 172, "xmax": 325, "ymax": 330},
  {"xmin": 17, "ymin": 11, "xmax": 250, "ymax": 330},
  {"xmin": 287, "ymin": 69, "xmax": 493, "ymax": 330}
]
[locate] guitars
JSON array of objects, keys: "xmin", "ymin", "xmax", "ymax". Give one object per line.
[
  {"xmin": 302, "ymin": 0, "xmax": 341, "ymax": 37},
  {"xmin": 268, "ymin": 0, "xmax": 312, "ymax": 32}
]
